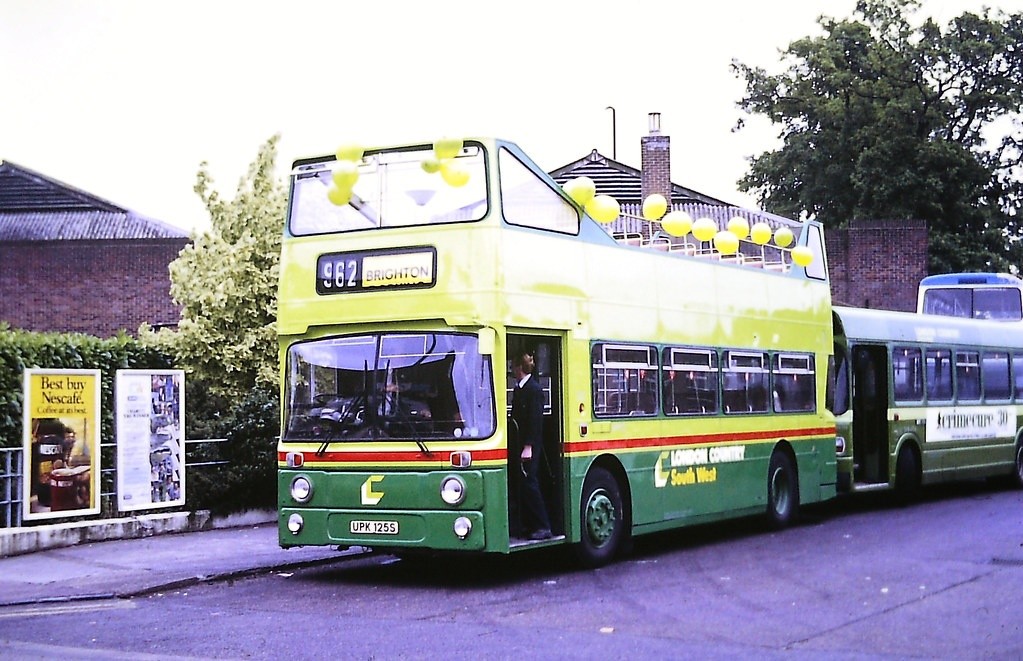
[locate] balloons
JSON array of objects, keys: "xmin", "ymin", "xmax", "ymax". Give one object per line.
[
  {"xmin": 750, "ymin": 223, "xmax": 772, "ymax": 244},
  {"xmin": 327, "ymin": 141, "xmax": 365, "ymax": 205},
  {"xmin": 791, "ymin": 246, "xmax": 813, "ymax": 266},
  {"xmin": 642, "ymin": 194, "xmax": 667, "ymax": 219},
  {"xmin": 692, "ymin": 218, "xmax": 717, "ymax": 241},
  {"xmin": 661, "ymin": 212, "xmax": 692, "ymax": 236},
  {"xmin": 562, "ymin": 176, "xmax": 620, "ymax": 223},
  {"xmin": 714, "ymin": 231, "xmax": 739, "ymax": 254},
  {"xmin": 728, "ymin": 217, "xmax": 749, "ymax": 239},
  {"xmin": 774, "ymin": 227, "xmax": 793, "ymax": 247},
  {"xmin": 422, "ymin": 137, "xmax": 471, "ymax": 186}
]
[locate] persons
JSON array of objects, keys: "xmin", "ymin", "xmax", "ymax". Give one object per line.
[
  {"xmin": 377, "ymin": 382, "xmax": 432, "ymax": 419},
  {"xmin": 509, "ymin": 353, "xmax": 552, "ymax": 539}
]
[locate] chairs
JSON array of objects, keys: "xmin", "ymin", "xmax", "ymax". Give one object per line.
[
  {"xmin": 601, "ymin": 381, "xmax": 748, "ymax": 414},
  {"xmin": 611, "ymin": 231, "xmax": 791, "ymax": 276}
]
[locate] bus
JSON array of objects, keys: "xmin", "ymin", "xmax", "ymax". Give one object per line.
[{"xmin": 273, "ymin": 133, "xmax": 1022, "ymax": 567}]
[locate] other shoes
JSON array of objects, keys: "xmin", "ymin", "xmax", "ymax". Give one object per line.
[{"xmin": 527, "ymin": 528, "xmax": 552, "ymax": 540}]
